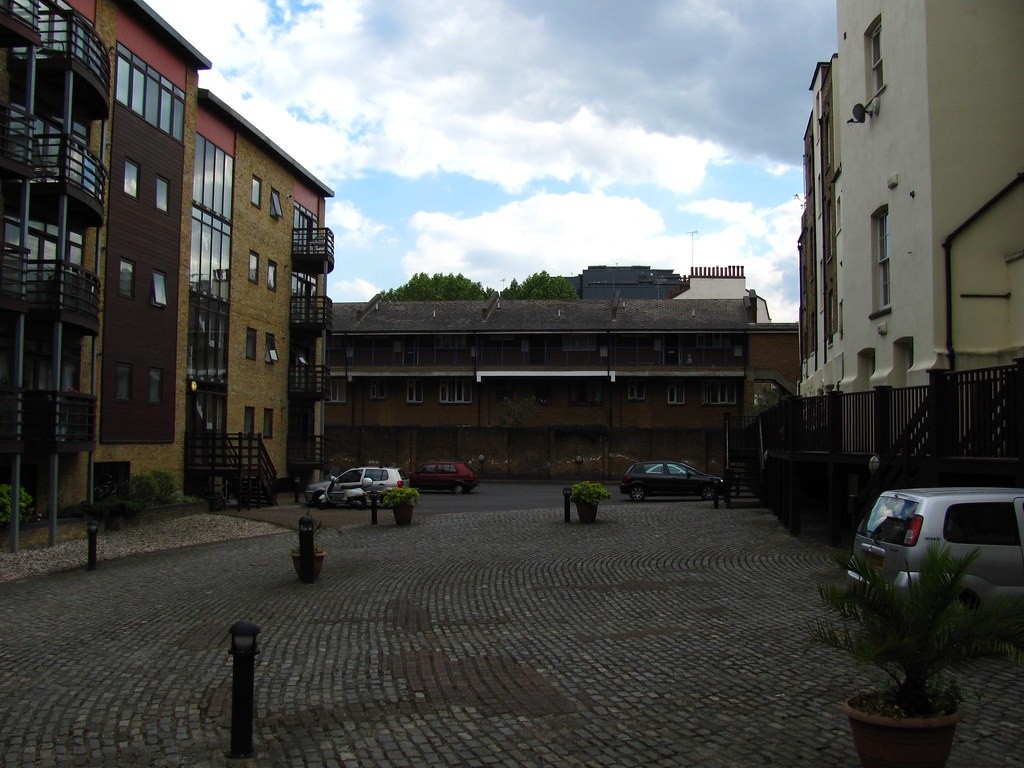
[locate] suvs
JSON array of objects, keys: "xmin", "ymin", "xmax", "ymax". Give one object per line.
[{"xmin": 844, "ymin": 488, "xmax": 1024, "ymax": 637}]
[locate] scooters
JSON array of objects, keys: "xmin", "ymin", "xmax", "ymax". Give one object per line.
[{"xmin": 317, "ymin": 473, "xmax": 373, "ymax": 511}]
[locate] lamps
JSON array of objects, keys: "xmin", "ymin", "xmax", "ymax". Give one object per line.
[
  {"xmin": 869, "ymin": 455, "xmax": 879, "ymax": 474},
  {"xmin": 191, "ymin": 380, "xmax": 197, "ymax": 392}
]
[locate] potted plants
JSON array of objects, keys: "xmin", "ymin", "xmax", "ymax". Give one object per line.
[
  {"xmin": 39, "ymin": 265, "xmax": 56, "ymax": 293},
  {"xmin": 801, "ymin": 537, "xmax": 1024, "ymax": 768},
  {"xmin": 378, "ymin": 487, "xmax": 420, "ymax": 525},
  {"xmin": 101, "ymin": 468, "xmax": 209, "ymax": 532},
  {"xmin": 289, "ymin": 507, "xmax": 326, "ymax": 579},
  {"xmin": 569, "ymin": 481, "xmax": 611, "ymax": 522},
  {"xmin": 0, "ymin": 484, "xmax": 109, "ymax": 550}
]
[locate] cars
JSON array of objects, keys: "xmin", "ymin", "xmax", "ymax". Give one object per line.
[
  {"xmin": 619, "ymin": 460, "xmax": 722, "ymax": 503},
  {"xmin": 403, "ymin": 460, "xmax": 479, "ymax": 495},
  {"xmin": 305, "ymin": 463, "xmax": 410, "ymax": 508}
]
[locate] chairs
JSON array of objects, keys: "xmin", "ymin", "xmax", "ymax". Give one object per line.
[{"xmin": 31, "ymin": 139, "xmax": 56, "ymax": 183}]
